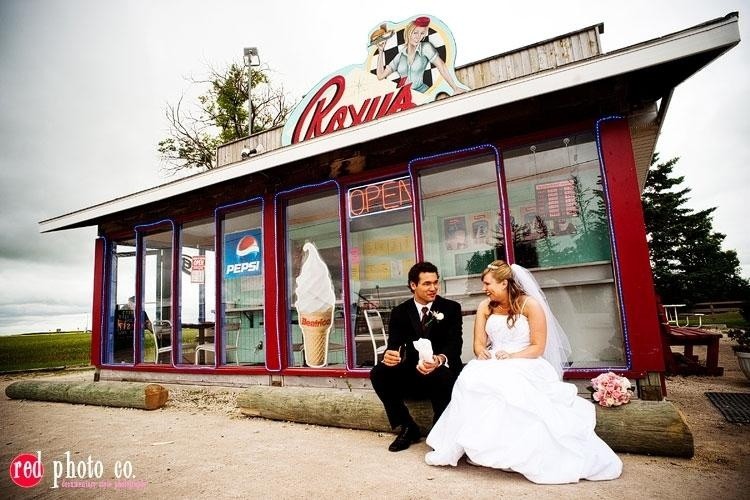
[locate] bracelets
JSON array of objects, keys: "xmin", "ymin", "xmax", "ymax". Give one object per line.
[{"xmin": 437, "ymin": 356, "xmax": 442, "ymax": 368}]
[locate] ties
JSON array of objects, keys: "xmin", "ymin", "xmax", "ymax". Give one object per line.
[{"xmin": 421, "ymin": 307, "xmax": 429, "ymax": 332}]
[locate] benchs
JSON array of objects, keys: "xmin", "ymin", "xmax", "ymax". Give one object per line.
[
  {"xmin": 679, "ymin": 313, "xmax": 704, "ymax": 329},
  {"xmin": 653, "ymin": 293, "xmax": 719, "ymax": 371}
]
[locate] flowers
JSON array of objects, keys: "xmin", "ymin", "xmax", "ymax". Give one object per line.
[{"xmin": 586, "ymin": 371, "xmax": 636, "ymax": 408}]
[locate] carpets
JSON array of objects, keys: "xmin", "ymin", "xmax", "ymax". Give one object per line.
[{"xmin": 704, "ymin": 390, "xmax": 750, "ymax": 423}]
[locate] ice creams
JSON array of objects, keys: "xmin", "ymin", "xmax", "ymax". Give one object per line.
[{"xmin": 295, "ymin": 243, "xmax": 335, "ymax": 366}]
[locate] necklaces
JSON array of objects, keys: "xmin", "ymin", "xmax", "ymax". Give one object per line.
[{"xmin": 498, "ymin": 304, "xmax": 508, "ymax": 312}]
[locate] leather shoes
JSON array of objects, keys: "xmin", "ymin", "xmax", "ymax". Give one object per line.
[{"xmin": 388, "ymin": 423, "xmax": 421, "ymax": 452}]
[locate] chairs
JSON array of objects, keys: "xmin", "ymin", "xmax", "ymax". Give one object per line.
[{"xmin": 151, "ymin": 308, "xmax": 388, "ymax": 367}]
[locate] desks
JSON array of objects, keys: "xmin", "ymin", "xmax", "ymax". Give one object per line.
[{"xmin": 661, "ymin": 304, "xmax": 685, "ymax": 327}]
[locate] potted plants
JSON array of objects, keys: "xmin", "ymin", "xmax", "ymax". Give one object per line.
[{"xmin": 726, "ymin": 298, "xmax": 750, "ymax": 381}]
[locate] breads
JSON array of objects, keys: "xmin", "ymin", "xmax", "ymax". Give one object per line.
[{"xmin": 370, "ymin": 29, "xmax": 385, "ymax": 41}]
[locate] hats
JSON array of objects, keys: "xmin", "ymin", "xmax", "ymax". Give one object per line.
[{"xmin": 415, "ymin": 16, "xmax": 431, "ymax": 28}]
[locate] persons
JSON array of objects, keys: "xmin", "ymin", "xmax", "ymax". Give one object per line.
[
  {"xmin": 377, "ymin": 17, "xmax": 467, "ymax": 94},
  {"xmin": 465, "ymin": 260, "xmax": 546, "ymax": 472},
  {"xmin": 370, "ymin": 262, "xmax": 464, "ymax": 452}
]
